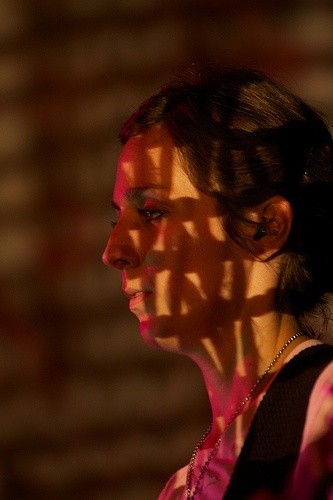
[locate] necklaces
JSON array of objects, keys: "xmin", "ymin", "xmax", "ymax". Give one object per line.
[{"xmin": 185, "ymin": 332, "xmax": 308, "ymax": 500}]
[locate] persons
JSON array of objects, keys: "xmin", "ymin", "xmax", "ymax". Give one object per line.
[{"xmin": 100, "ymin": 63, "xmax": 333, "ymax": 500}]
